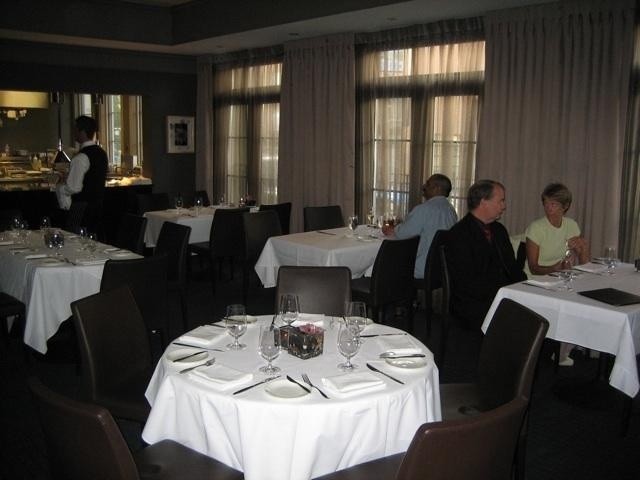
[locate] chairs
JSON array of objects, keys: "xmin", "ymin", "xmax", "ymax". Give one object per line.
[{"xmin": 2, "ymin": 190, "xmax": 547, "ymax": 480}]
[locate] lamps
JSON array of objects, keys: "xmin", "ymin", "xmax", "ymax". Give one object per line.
[{"xmin": 51, "ymin": 92, "xmax": 72, "ymax": 164}]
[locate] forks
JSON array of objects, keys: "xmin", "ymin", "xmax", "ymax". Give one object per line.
[
  {"xmin": 302, "ymin": 372, "xmax": 332, "ymax": 401},
  {"xmin": 379, "ymin": 351, "xmax": 426, "ymax": 359},
  {"xmin": 179, "ymin": 357, "xmax": 215, "ymax": 375}
]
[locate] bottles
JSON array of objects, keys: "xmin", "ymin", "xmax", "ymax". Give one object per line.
[{"xmin": 4, "ymin": 143, "xmax": 10, "ymax": 156}]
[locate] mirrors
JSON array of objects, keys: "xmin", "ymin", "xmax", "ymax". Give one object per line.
[{"xmin": 0, "ymin": 88, "xmax": 154, "ymax": 192}]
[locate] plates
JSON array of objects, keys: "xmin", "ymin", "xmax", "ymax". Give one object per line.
[
  {"xmin": 162, "ymin": 348, "xmax": 210, "ymax": 366},
  {"xmin": 102, "ymin": 247, "xmax": 130, "ymax": 257},
  {"xmin": 25, "ymin": 170, "xmax": 42, "ymax": 176},
  {"xmin": 263, "ymin": 377, "xmax": 311, "ymax": 400},
  {"xmin": 385, "ymin": 353, "xmax": 428, "ymax": 370}
]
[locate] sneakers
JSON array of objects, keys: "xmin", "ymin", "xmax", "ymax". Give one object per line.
[{"xmin": 559, "ymin": 357, "xmax": 574, "ymax": 366}]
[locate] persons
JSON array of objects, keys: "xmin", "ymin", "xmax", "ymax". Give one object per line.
[
  {"xmin": 384, "ymin": 174, "xmax": 458, "ymax": 289},
  {"xmin": 53, "ymin": 115, "xmax": 108, "ymax": 243},
  {"xmin": 445, "ymin": 179, "xmax": 526, "ymax": 302},
  {"xmin": 520, "ymin": 182, "xmax": 591, "ymax": 367}
]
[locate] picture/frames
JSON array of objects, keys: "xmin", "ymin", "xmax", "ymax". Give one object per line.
[{"xmin": 165, "ymin": 114, "xmax": 196, "ymax": 153}]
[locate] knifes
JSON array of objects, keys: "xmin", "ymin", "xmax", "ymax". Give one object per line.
[
  {"xmin": 172, "ymin": 350, "xmax": 209, "ymax": 362},
  {"xmin": 365, "ymin": 363, "xmax": 406, "ymax": 387},
  {"xmin": 285, "ymin": 373, "xmax": 312, "ymax": 394},
  {"xmin": 360, "ymin": 333, "xmax": 408, "ymax": 338},
  {"xmin": 172, "ymin": 342, "xmax": 222, "ymax": 352}
]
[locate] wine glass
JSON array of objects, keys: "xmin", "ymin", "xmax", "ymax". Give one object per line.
[
  {"xmin": 219, "ymin": 289, "xmax": 370, "ymax": 379},
  {"xmin": 9, "ymin": 216, "xmax": 97, "ymax": 262},
  {"xmin": 555, "ymin": 246, "xmax": 616, "ymax": 292},
  {"xmin": 168, "ymin": 191, "xmax": 247, "ymax": 220},
  {"xmin": 346, "ymin": 209, "xmax": 399, "ymax": 243}
]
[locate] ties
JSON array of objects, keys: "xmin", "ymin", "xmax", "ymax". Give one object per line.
[{"xmin": 484, "ymin": 228, "xmax": 492, "ymax": 241}]
